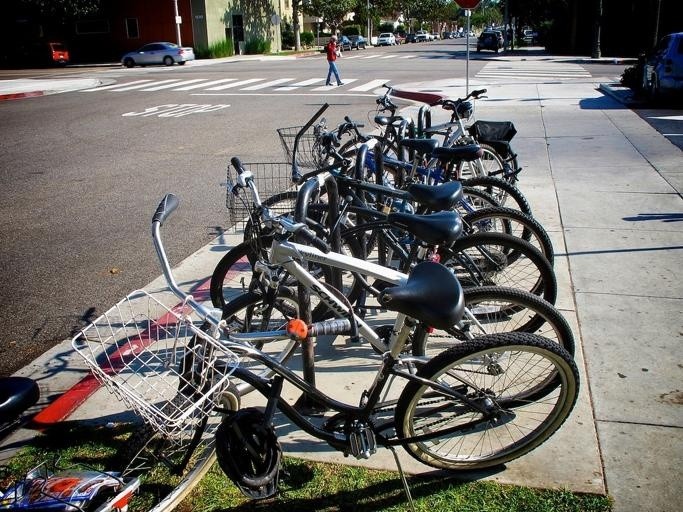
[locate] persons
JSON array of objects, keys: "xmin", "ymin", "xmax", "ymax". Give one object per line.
[{"xmin": 325, "ymin": 36, "xmax": 343, "ymax": 86}]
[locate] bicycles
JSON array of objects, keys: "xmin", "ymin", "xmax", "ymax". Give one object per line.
[
  {"xmin": 69, "ymin": 194, "xmax": 579, "ymax": 510},
  {"xmin": 180, "ymin": 156, "xmax": 577, "ymax": 407},
  {"xmin": 223, "ymin": 83, "xmax": 557, "ymax": 344},
  {"xmin": 0, "ymin": 374, "xmax": 143, "ymax": 512}
]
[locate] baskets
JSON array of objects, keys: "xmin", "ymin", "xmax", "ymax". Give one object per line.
[
  {"xmin": 279, "ymin": 127, "xmax": 318, "ymax": 166},
  {"xmin": 71, "ymin": 289, "xmax": 240, "ymax": 448},
  {"xmin": 226, "ymin": 162, "xmax": 299, "ymax": 230}
]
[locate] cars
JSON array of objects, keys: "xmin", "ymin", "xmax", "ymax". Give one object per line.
[
  {"xmin": 483, "ymin": 26, "xmax": 515, "ymax": 48},
  {"xmin": 120, "ymin": 42, "xmax": 196, "ymax": 65},
  {"xmin": 377, "ymin": 33, "xmax": 397, "ymax": 46},
  {"xmin": 393, "ymin": 34, "xmax": 402, "ymax": 45},
  {"xmin": 404, "ymin": 30, "xmax": 474, "ymax": 43},
  {"xmin": 323, "ymin": 35, "xmax": 353, "ymax": 53},
  {"xmin": 348, "ymin": 35, "xmax": 368, "ymax": 50}
]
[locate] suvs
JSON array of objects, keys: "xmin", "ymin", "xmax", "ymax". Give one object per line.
[
  {"xmin": 477, "ymin": 32, "xmax": 501, "ymax": 53},
  {"xmin": 641, "ymin": 32, "xmax": 683, "ymax": 102}
]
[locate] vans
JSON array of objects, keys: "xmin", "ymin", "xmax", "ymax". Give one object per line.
[{"xmin": 45, "ymin": 40, "xmax": 70, "ymax": 67}]
